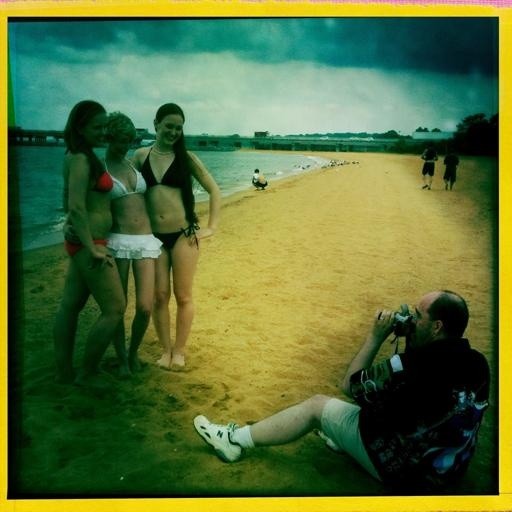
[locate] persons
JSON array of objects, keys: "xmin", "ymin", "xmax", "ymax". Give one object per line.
[
  {"xmin": 192, "ymin": 287, "xmax": 492, "ymax": 489},
  {"xmin": 131, "ymin": 102, "xmax": 224, "ymax": 371},
  {"xmin": 252, "ymin": 169, "xmax": 268, "ymax": 191},
  {"xmin": 443, "ymin": 147, "xmax": 460, "ymax": 190},
  {"xmin": 418, "ymin": 142, "xmax": 439, "ymax": 190},
  {"xmin": 64, "ymin": 110, "xmax": 165, "ymax": 380},
  {"xmin": 53, "ymin": 99, "xmax": 127, "ymax": 392}
]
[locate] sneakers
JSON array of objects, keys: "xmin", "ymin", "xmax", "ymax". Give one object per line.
[
  {"xmin": 194, "ymin": 414, "xmax": 244, "ymax": 463},
  {"xmin": 311, "ymin": 427, "xmax": 344, "ymax": 453}
]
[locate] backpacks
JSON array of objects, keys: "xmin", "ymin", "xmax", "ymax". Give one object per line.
[{"xmin": 259, "ymin": 175, "xmax": 266, "ymax": 184}]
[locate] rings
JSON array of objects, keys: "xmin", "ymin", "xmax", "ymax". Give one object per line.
[{"xmin": 380, "ymin": 317, "xmax": 385, "ymax": 320}]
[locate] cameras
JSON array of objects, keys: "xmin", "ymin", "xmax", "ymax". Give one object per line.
[{"xmin": 378, "ymin": 303, "xmax": 416, "ymax": 338}]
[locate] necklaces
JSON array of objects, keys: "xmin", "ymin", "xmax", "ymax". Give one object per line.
[{"xmin": 154, "ymin": 146, "xmax": 174, "ymax": 155}]
